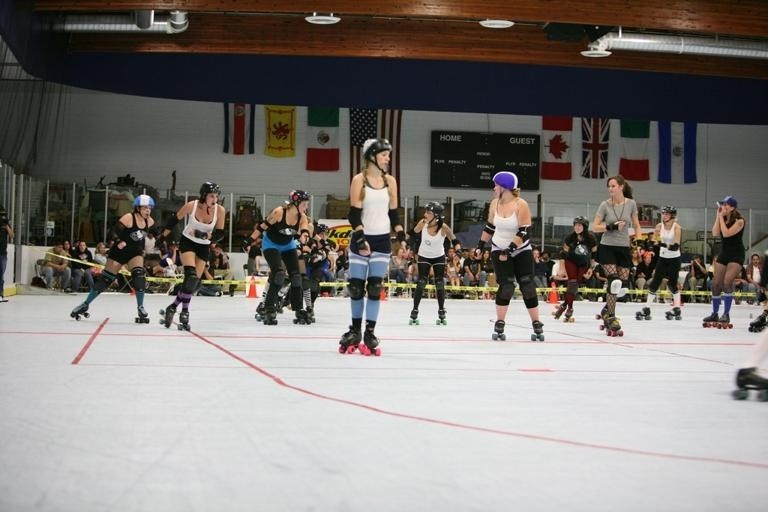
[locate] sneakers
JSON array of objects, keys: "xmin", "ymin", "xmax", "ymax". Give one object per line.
[
  {"xmin": 748, "ymin": 316, "xmax": 766, "ymax": 333},
  {"xmin": 733, "ymin": 367, "xmax": 768, "ymax": 402}
]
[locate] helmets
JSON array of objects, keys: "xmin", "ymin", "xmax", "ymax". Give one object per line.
[
  {"xmin": 661, "ymin": 205, "xmax": 676, "ymax": 214},
  {"xmin": 363, "ymin": 138, "xmax": 392, "ymax": 160},
  {"xmin": 492, "ymin": 172, "xmax": 518, "ymax": 191},
  {"xmin": 133, "ymin": 193, "xmax": 155, "ymax": 207},
  {"xmin": 289, "ymin": 190, "xmax": 310, "ymax": 207},
  {"xmin": 199, "ymin": 182, "xmax": 221, "ymax": 195},
  {"xmin": 573, "ymin": 214, "xmax": 590, "ymax": 228},
  {"xmin": 425, "ymin": 202, "xmax": 444, "ymax": 215}
]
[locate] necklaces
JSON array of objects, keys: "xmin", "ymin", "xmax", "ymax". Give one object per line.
[{"xmin": 611, "ymin": 197, "xmax": 625, "ymax": 221}]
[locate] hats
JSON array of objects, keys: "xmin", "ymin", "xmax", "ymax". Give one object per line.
[{"xmin": 719, "ymin": 196, "xmax": 738, "ymax": 209}]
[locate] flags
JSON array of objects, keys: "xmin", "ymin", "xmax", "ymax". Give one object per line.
[
  {"xmin": 654, "ymin": 119, "xmax": 698, "ymax": 184},
  {"xmin": 305, "ymin": 106, "xmax": 340, "ymax": 172},
  {"xmin": 348, "ymin": 107, "xmax": 402, "ymax": 210},
  {"xmin": 221, "ymin": 102, "xmax": 255, "ymax": 155},
  {"xmin": 579, "ymin": 117, "xmax": 610, "ymax": 181},
  {"xmin": 617, "ymin": 117, "xmax": 651, "ymax": 182},
  {"xmin": 539, "ymin": 115, "xmax": 572, "ymax": 181},
  {"xmin": 262, "ymin": 104, "xmax": 297, "ymax": 159}
]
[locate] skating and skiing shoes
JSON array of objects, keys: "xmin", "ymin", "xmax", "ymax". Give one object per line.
[
  {"xmin": 361, "ymin": 327, "xmax": 381, "ymax": 356},
  {"xmin": 338, "ymin": 325, "xmax": 361, "ymax": 354},
  {"xmin": 491, "ymin": 319, "xmax": 506, "ymax": 340},
  {"xmin": 635, "ymin": 308, "xmax": 651, "ymax": 320},
  {"xmin": 702, "ymin": 313, "xmax": 733, "ymax": 329},
  {"xmin": 70, "ymin": 301, "xmax": 91, "ymax": 321},
  {"xmin": 409, "ymin": 309, "xmax": 419, "ymax": 325},
  {"xmin": 596, "ymin": 305, "xmax": 624, "ymax": 338},
  {"xmin": 135, "ymin": 304, "xmax": 151, "ymax": 324},
  {"xmin": 159, "ymin": 304, "xmax": 177, "ymax": 328},
  {"xmin": 552, "ymin": 303, "xmax": 574, "ymax": 323},
  {"xmin": 177, "ymin": 312, "xmax": 191, "ymax": 330},
  {"xmin": 437, "ymin": 310, "xmax": 447, "ymax": 325},
  {"xmin": 665, "ymin": 307, "xmax": 681, "ymax": 320},
  {"xmin": 531, "ymin": 320, "xmax": 545, "ymax": 340},
  {"xmin": 255, "ymin": 296, "xmax": 315, "ymax": 325}
]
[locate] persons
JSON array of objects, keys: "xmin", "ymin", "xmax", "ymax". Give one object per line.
[
  {"xmin": 408, "ymin": 202, "xmax": 463, "ymax": 326},
  {"xmin": 474, "ymin": 171, "xmax": 551, "ymax": 347},
  {"xmin": 700, "ymin": 193, "xmax": 744, "ymax": 331},
  {"xmin": 156, "ymin": 181, "xmax": 226, "ymax": 331},
  {"xmin": 334, "ymin": 133, "xmax": 402, "ymax": 356},
  {"xmin": 589, "ymin": 171, "xmax": 644, "ymax": 340},
  {"xmin": 748, "ymin": 301, "xmax": 768, "ymax": 332},
  {"xmin": 246, "ymin": 216, "xmax": 768, "ymax": 322},
  {"xmin": 69, "ymin": 193, "xmax": 160, "ymax": 326},
  {"xmin": 0, "ymin": 204, "xmax": 12, "ymax": 304},
  {"xmin": 40, "ymin": 224, "xmax": 238, "ymax": 297},
  {"xmin": 242, "ymin": 190, "xmax": 315, "ymax": 326}
]
